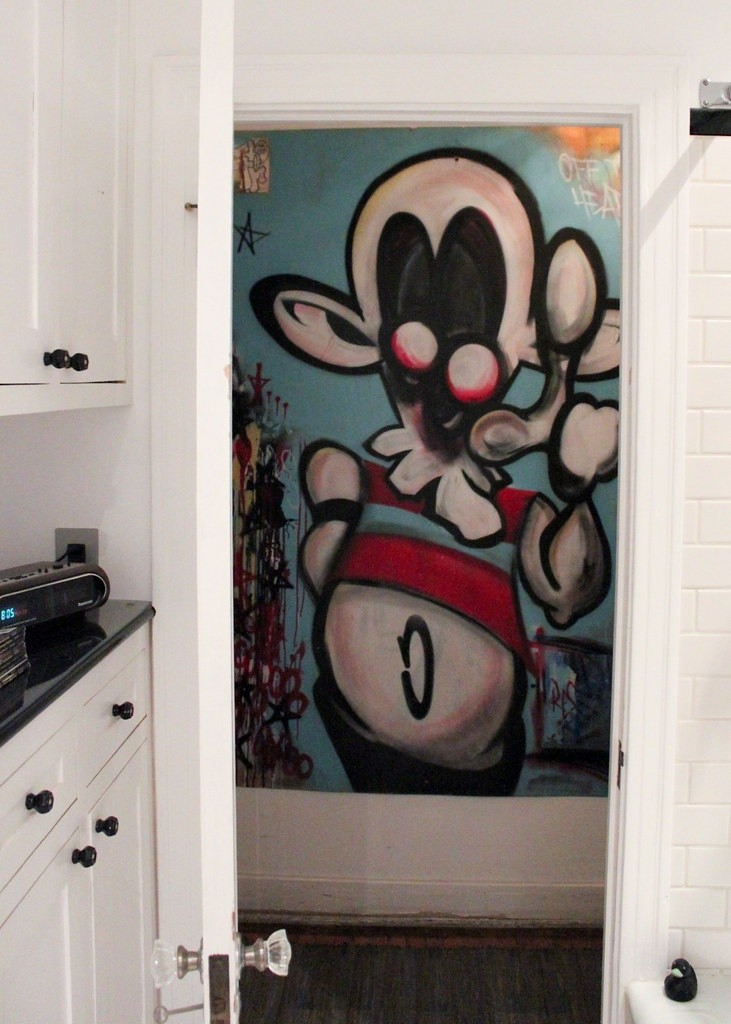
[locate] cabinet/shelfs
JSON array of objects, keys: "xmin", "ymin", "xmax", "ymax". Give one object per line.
[{"xmin": 0, "ymin": 0, "xmax": 163, "ymax": 1024}]
